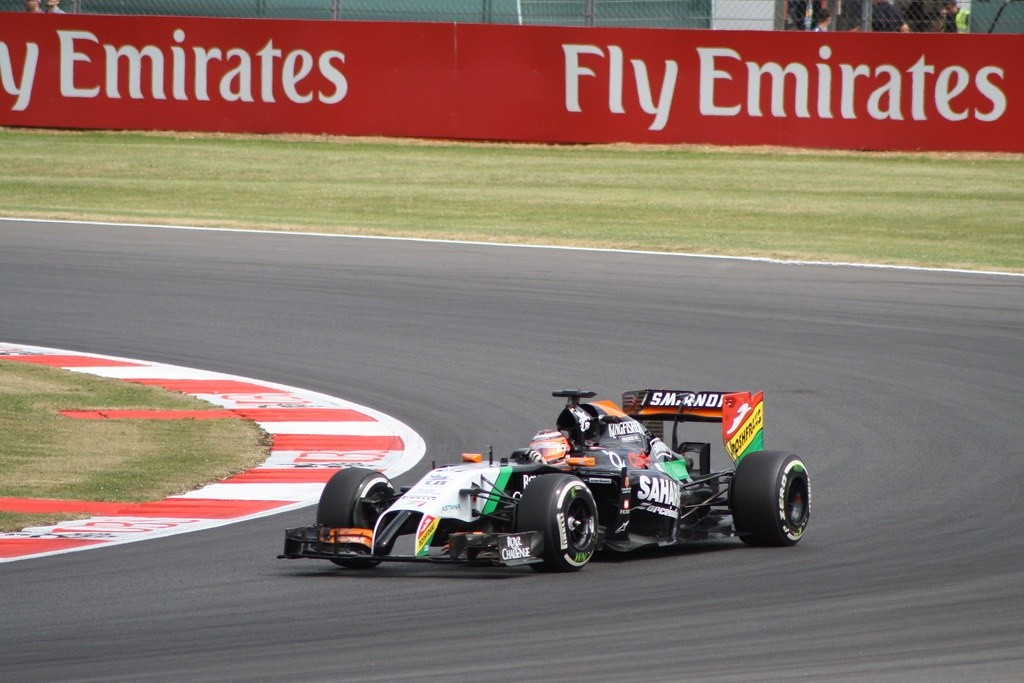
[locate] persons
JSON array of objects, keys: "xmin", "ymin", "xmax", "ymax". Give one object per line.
[
  {"xmin": 782, "ymin": 0, "xmax": 971, "ymax": 33},
  {"xmin": 529, "ymin": 427, "xmax": 574, "ymax": 468}
]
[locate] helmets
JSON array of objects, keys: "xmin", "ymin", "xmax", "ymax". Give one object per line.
[{"xmin": 530, "ymin": 430, "xmax": 571, "ymax": 462}]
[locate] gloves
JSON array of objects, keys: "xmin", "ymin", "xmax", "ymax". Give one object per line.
[{"xmin": 526, "ymin": 450, "xmax": 547, "ymax": 465}]
